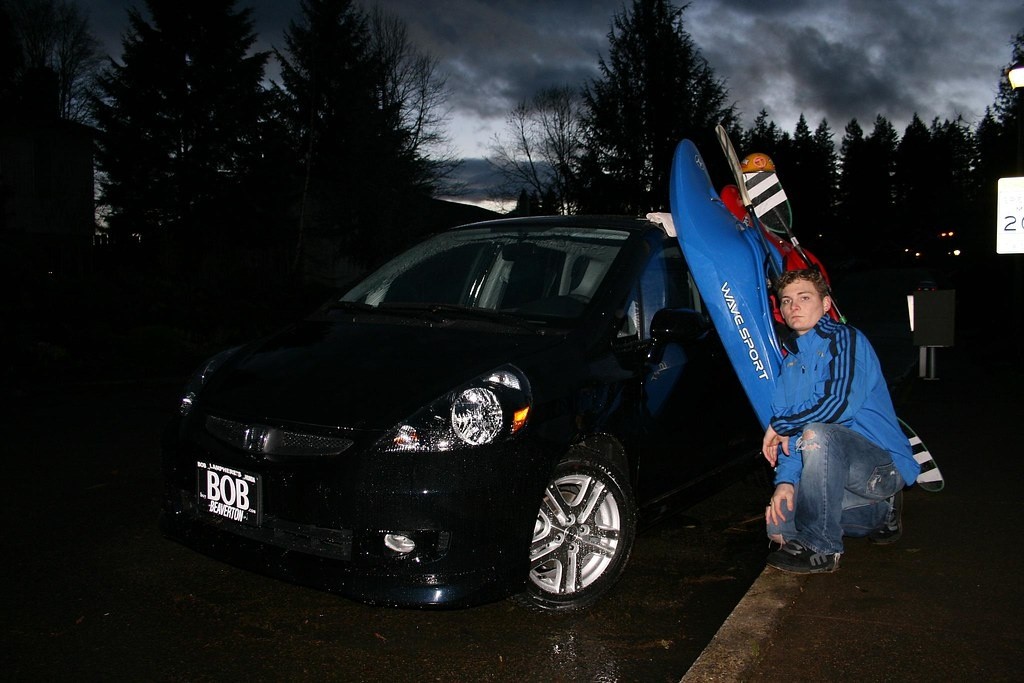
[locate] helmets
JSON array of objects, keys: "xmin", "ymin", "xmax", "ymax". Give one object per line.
[{"xmin": 740, "ymin": 152, "xmax": 776, "ymax": 174}]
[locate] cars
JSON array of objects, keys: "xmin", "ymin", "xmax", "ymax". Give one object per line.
[{"xmin": 164, "ymin": 210, "xmax": 770, "ymax": 620}]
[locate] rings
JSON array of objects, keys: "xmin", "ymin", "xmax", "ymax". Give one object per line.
[{"xmin": 767, "ymin": 502, "xmax": 772, "ymax": 507}]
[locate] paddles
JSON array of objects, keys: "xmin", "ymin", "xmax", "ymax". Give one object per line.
[
  {"xmin": 714, "ymin": 123, "xmax": 784, "ymax": 284},
  {"xmin": 740, "ymin": 153, "xmax": 943, "ymax": 496}
]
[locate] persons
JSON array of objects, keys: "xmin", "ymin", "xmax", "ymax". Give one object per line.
[{"xmin": 761, "ymin": 267, "xmax": 920, "ymax": 575}]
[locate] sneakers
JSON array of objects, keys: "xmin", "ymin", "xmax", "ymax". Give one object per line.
[
  {"xmin": 766, "ymin": 537, "xmax": 840, "ymax": 576},
  {"xmin": 871, "ymin": 488, "xmax": 904, "ymax": 545}
]
